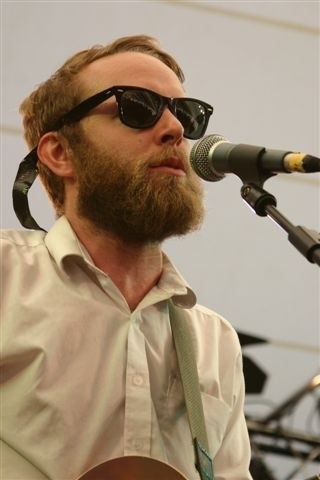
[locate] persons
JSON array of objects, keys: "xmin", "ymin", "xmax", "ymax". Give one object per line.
[{"xmin": 1, "ymin": 34, "xmax": 257, "ymax": 480}]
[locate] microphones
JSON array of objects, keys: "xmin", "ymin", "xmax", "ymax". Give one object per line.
[{"xmin": 189, "ymin": 133, "xmax": 320, "ymax": 182}]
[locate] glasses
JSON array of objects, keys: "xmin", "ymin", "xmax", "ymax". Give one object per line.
[{"xmin": 50, "ymin": 85, "xmax": 214, "ymax": 140}]
